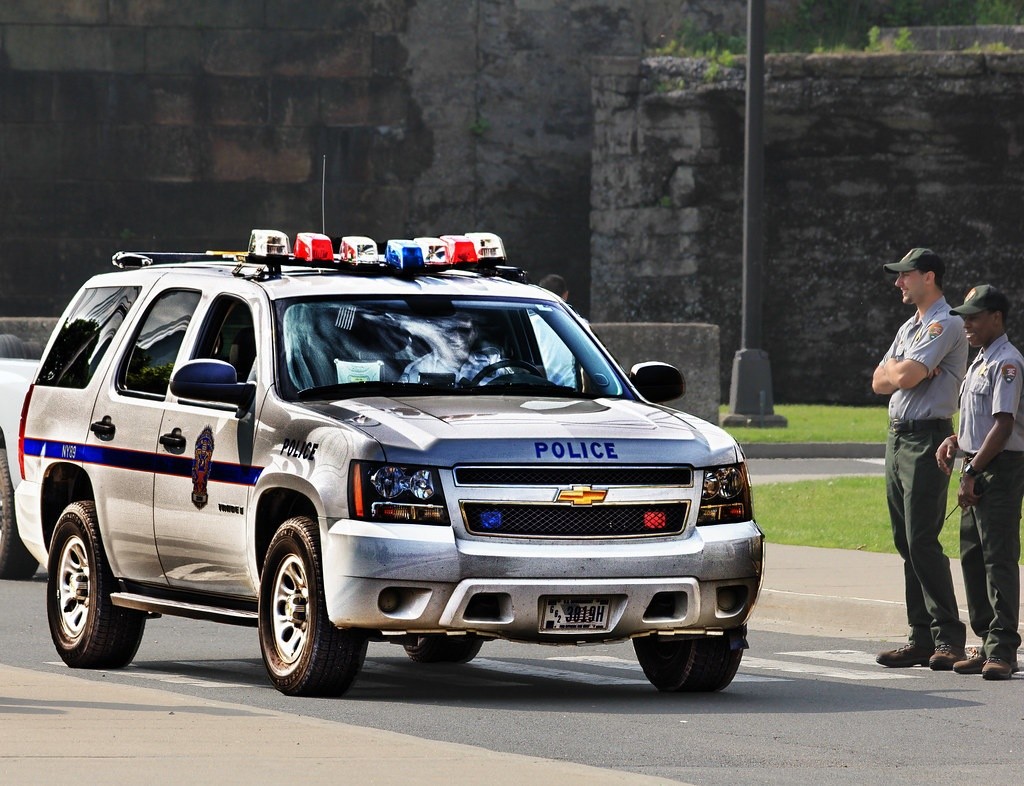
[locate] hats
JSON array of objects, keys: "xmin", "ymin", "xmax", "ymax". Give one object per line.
[
  {"xmin": 883, "ymin": 248, "xmax": 945, "ymax": 278},
  {"xmin": 949, "ymin": 285, "xmax": 1008, "ymax": 316}
]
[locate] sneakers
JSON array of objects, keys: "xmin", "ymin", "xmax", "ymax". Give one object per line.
[
  {"xmin": 929, "ymin": 645, "xmax": 967, "ymax": 669},
  {"xmin": 983, "ymin": 658, "xmax": 1012, "ymax": 680},
  {"xmin": 953, "ymin": 656, "xmax": 985, "ymax": 674},
  {"xmin": 876, "ymin": 644, "xmax": 933, "ymax": 668}
]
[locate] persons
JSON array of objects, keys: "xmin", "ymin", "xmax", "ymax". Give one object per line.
[
  {"xmin": 933, "ymin": 284, "xmax": 1024, "ymax": 675},
  {"xmin": 871, "ymin": 248, "xmax": 969, "ymax": 672},
  {"xmin": 539, "ymin": 274, "xmax": 568, "ymax": 302},
  {"xmin": 403, "ymin": 310, "xmax": 513, "ymax": 389}
]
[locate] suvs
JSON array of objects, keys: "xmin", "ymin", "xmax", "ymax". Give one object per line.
[{"xmin": 11, "ymin": 227, "xmax": 765, "ymax": 700}]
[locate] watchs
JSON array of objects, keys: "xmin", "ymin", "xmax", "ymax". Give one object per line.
[{"xmin": 964, "ymin": 464, "xmax": 976, "ymax": 477}]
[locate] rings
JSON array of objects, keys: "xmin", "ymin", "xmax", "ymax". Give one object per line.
[{"xmin": 962, "ymin": 501, "xmax": 965, "ymax": 504}]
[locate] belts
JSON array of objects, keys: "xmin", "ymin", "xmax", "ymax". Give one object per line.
[
  {"xmin": 889, "ymin": 418, "xmax": 951, "ymax": 431},
  {"xmin": 967, "ymin": 450, "xmax": 1024, "ymax": 462}
]
